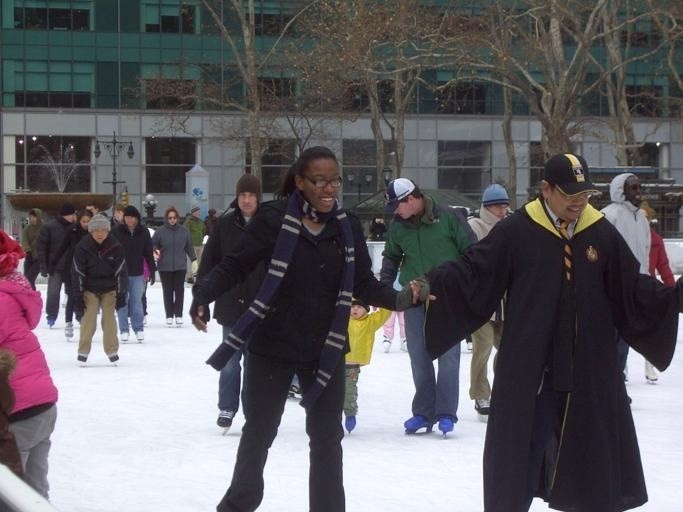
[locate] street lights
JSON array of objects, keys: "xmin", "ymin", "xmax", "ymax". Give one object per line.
[
  {"xmin": 90, "ymin": 129, "xmax": 136, "ymax": 206},
  {"xmin": 347, "ymin": 171, "xmax": 372, "ymax": 200},
  {"xmin": 379, "ymin": 166, "xmax": 393, "ymax": 188}
]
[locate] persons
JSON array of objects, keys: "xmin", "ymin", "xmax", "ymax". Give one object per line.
[
  {"xmin": 188, "ymin": 145, "xmax": 421, "ymax": 512},
  {"xmin": 19, "ymin": 204, "xmax": 217, "ymax": 365},
  {"xmin": 0, "ymin": 228, "xmax": 58, "ymax": 502},
  {"xmin": 598, "ymin": 173, "xmax": 677, "ymax": 382},
  {"xmin": 287, "ymin": 181, "xmax": 515, "ymax": 438},
  {"xmin": 410, "ymin": 151, "xmax": 683, "ymax": 512},
  {"xmin": 192, "ymin": 172, "xmax": 263, "ymax": 437}
]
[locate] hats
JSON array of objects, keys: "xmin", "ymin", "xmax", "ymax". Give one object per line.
[
  {"xmin": 384, "ymin": 179, "xmax": 415, "ymax": 214},
  {"xmin": 481, "ymin": 184, "xmax": 509, "ymax": 206},
  {"xmin": 540, "ymin": 153, "xmax": 602, "ymax": 197},
  {"xmin": 235, "ymin": 172, "xmax": 262, "ymax": 200},
  {"xmin": 30, "ymin": 207, "xmax": 218, "ymax": 233}
]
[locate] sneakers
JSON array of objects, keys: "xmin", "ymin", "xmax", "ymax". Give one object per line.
[
  {"xmin": 217, "ymin": 410, "xmax": 234, "ymax": 427},
  {"xmin": 345, "ymin": 339, "xmax": 491, "ymax": 433},
  {"xmin": 48, "ymin": 316, "xmax": 184, "ymax": 362}
]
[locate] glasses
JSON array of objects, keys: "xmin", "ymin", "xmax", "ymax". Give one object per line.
[{"xmin": 301, "ymin": 173, "xmax": 342, "ymax": 188}]
